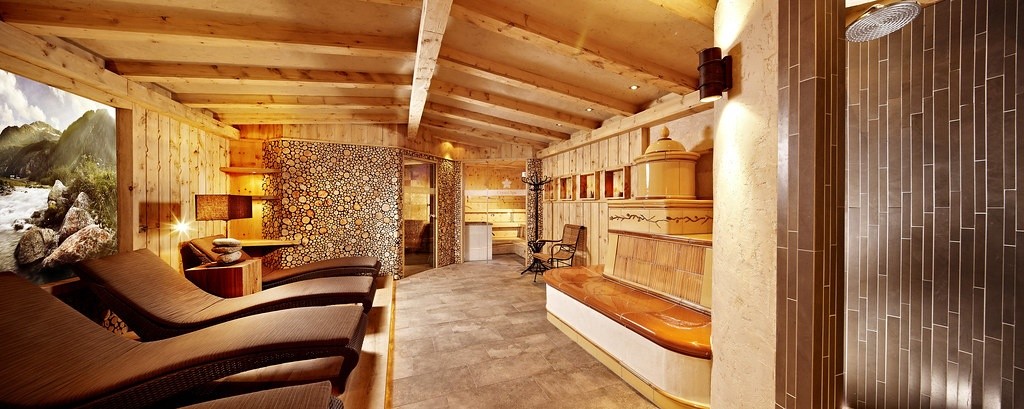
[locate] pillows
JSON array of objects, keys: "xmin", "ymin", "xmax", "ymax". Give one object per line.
[{"xmin": 100, "ymin": 309, "xmax": 140, "ymax": 339}]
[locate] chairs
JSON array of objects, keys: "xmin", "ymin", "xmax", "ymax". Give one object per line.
[
  {"xmin": 177, "ymin": 380, "xmax": 344, "ymax": 409},
  {"xmin": 531, "ymin": 224, "xmax": 584, "ymax": 269},
  {"xmin": 187, "ymin": 234, "xmax": 381, "ymax": 291},
  {"xmin": 0, "ymin": 271, "xmax": 368, "ymax": 409},
  {"xmin": 72, "ymin": 248, "xmax": 376, "ymax": 339}
]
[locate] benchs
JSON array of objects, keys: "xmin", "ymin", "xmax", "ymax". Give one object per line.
[{"xmin": 543, "ymin": 229, "xmax": 712, "ymax": 409}]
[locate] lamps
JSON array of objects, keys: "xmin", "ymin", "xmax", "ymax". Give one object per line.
[
  {"xmin": 195, "ymin": 194, "xmax": 253, "ymax": 237},
  {"xmin": 697, "ymin": 47, "xmax": 733, "ymax": 103}
]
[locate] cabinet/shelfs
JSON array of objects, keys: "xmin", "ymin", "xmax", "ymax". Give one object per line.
[{"xmin": 220, "ymin": 167, "xmax": 300, "ymax": 257}]
